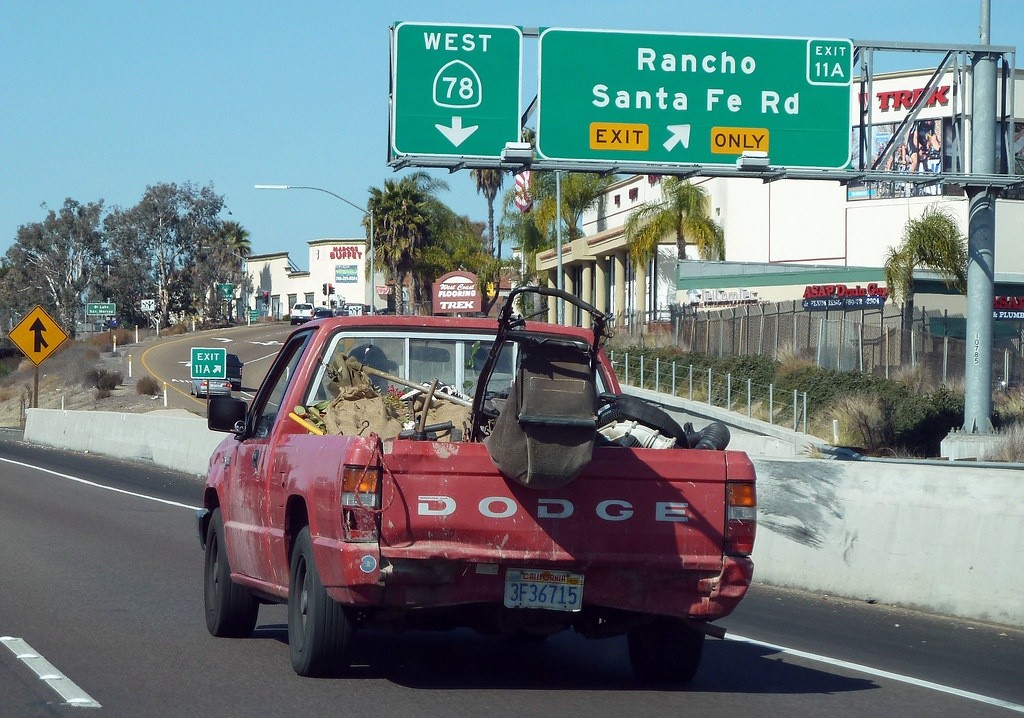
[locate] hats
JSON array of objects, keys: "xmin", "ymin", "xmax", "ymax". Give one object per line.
[{"xmin": 347, "ymin": 343, "xmax": 397, "ymax": 372}]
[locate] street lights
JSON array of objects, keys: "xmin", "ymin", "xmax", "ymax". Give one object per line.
[{"xmin": 254, "ymin": 184, "xmax": 374, "ymax": 317}]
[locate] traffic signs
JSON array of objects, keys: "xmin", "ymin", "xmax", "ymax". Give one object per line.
[{"xmin": 538, "ymin": 26, "xmax": 855, "ymax": 170}]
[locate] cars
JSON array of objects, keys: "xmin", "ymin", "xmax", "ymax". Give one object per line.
[
  {"xmin": 190, "ymin": 353, "xmax": 244, "ymax": 399},
  {"xmin": 289, "ymin": 302, "xmax": 390, "ymax": 326}
]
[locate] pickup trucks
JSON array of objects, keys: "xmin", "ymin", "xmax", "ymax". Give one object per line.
[{"xmin": 195, "ymin": 315, "xmax": 760, "ymax": 679}]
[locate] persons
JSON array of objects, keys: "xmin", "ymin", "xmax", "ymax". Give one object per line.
[
  {"xmin": 1014, "ymin": 123, "xmax": 1024, "ymax": 157},
  {"xmin": 885, "ymin": 120, "xmax": 941, "ymax": 173},
  {"xmin": 348, "ymin": 344, "xmax": 398, "ymax": 392}
]
